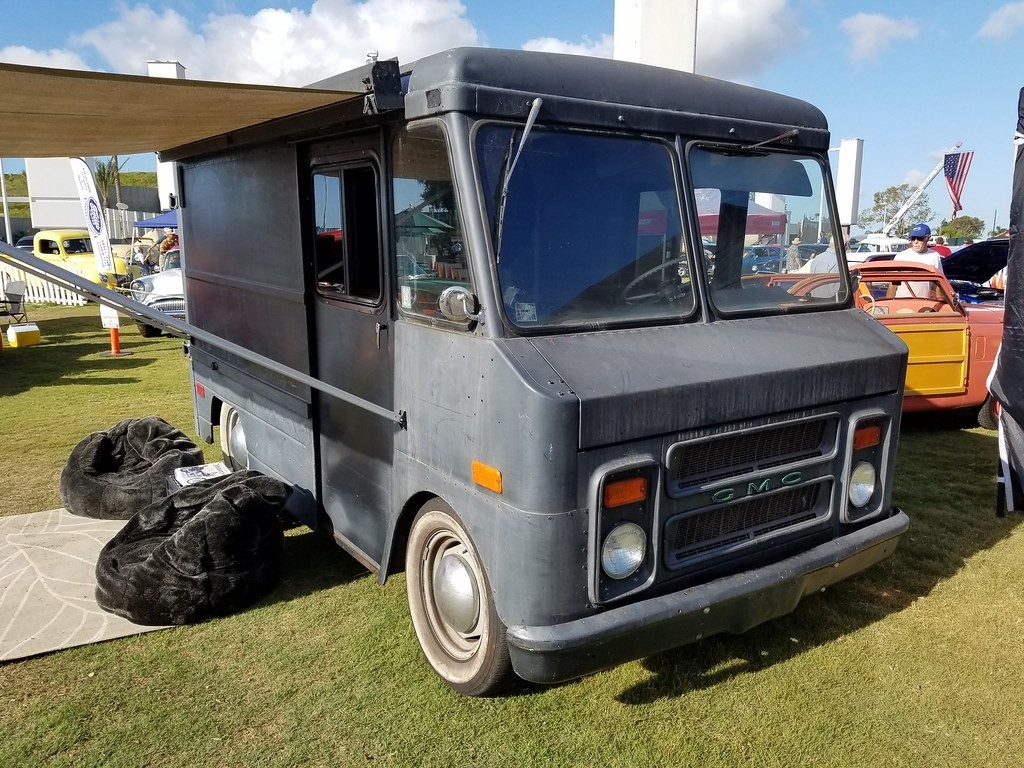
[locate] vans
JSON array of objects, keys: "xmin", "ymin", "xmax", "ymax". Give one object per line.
[{"xmin": 155, "ymin": 46, "xmax": 910, "ymax": 700}]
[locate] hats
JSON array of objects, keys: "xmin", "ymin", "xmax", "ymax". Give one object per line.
[
  {"xmin": 936, "ymin": 237, "xmax": 944, "ymax": 243},
  {"xmin": 791, "ymin": 238, "xmax": 801, "ymax": 245},
  {"xmin": 910, "ymin": 224, "xmax": 930, "ymax": 237}
]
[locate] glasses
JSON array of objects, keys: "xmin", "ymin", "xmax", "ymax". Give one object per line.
[{"xmin": 912, "ymin": 235, "xmax": 929, "ymax": 241}]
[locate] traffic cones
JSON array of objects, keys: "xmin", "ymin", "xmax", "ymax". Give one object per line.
[
  {"xmin": 448, "ymin": 267, "xmax": 456, "ymax": 280},
  {"xmin": 434, "ymin": 262, "xmax": 443, "ymax": 278},
  {"xmin": 442, "ymin": 264, "xmax": 450, "ymax": 279},
  {"xmin": 456, "ymin": 269, "xmax": 463, "ymax": 282}
]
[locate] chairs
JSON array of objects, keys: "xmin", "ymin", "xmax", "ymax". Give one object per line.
[{"xmin": 0, "ymin": 281, "xmax": 28, "ymax": 323}]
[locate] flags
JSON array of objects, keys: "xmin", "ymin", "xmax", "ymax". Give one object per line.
[{"xmin": 944, "ymin": 151, "xmax": 974, "ymax": 221}]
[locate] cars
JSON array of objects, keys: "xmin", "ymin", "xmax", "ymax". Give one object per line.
[
  {"xmin": 315, "ymin": 228, "xmax": 478, "ymax": 320},
  {"xmin": 12, "ymin": 227, "xmax": 181, "ymax": 305},
  {"xmin": 680, "ymin": 232, "xmax": 1011, "ymax": 430},
  {"xmin": 128, "ymin": 246, "xmax": 185, "ymax": 339}
]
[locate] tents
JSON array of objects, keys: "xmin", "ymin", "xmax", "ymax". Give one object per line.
[
  {"xmin": 637, "ymin": 188, "xmax": 788, "ymax": 282},
  {"xmin": 130, "ymin": 209, "xmax": 178, "ymax": 265}
]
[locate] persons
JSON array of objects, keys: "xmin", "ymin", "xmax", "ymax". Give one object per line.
[
  {"xmin": 451, "ymin": 240, "xmax": 463, "ymax": 263},
  {"xmin": 885, "ymin": 223, "xmax": 944, "ymax": 298},
  {"xmin": 923, "ymin": 236, "xmax": 974, "ymax": 257},
  {"xmin": 134, "ymin": 228, "xmax": 179, "ymax": 276},
  {"xmin": 786, "ymin": 230, "xmax": 850, "ymax": 298}
]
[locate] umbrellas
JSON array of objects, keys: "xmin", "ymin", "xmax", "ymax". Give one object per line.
[{"xmin": 395, "ymin": 204, "xmax": 455, "ymax": 261}]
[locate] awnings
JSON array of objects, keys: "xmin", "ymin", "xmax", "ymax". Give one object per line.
[
  {"xmin": 0, "ymin": 63, "xmax": 408, "ymax": 431},
  {"xmin": 315, "ymin": 138, "xmax": 453, "ymax": 182}
]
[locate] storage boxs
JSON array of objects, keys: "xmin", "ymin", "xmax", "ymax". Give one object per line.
[{"xmin": 7, "ymin": 322, "xmax": 41, "ymax": 348}]
